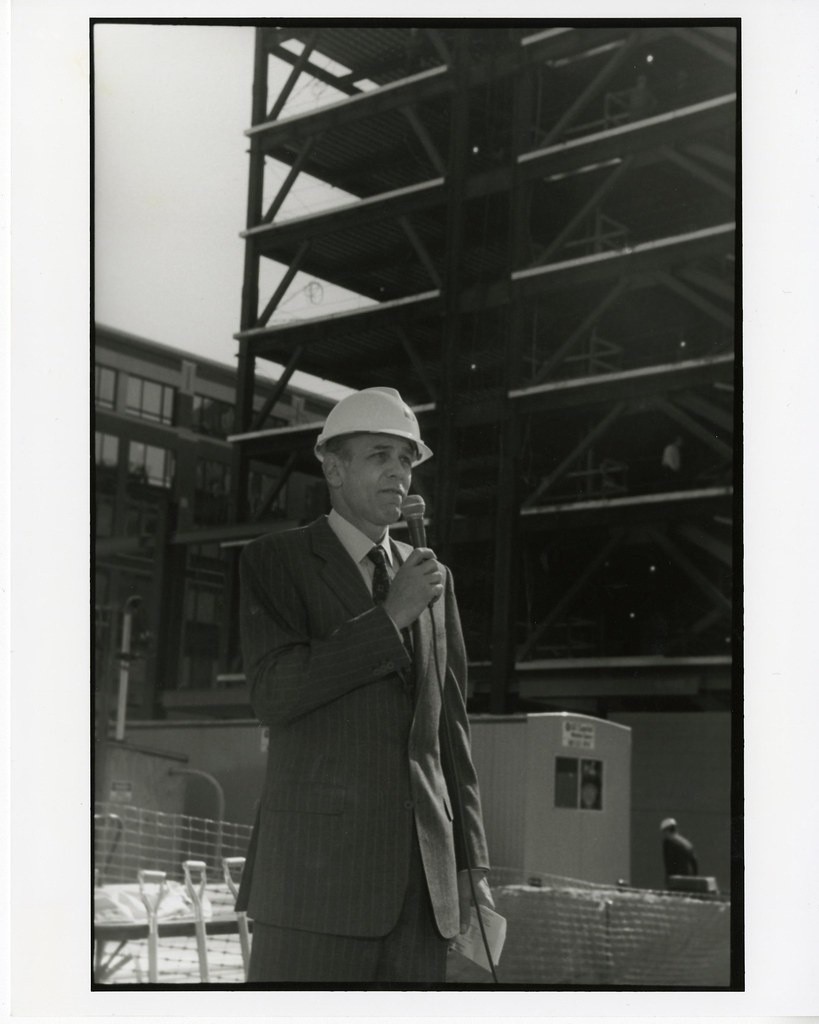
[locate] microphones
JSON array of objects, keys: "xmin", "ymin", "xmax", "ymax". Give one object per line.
[{"xmin": 399, "ymin": 495, "xmax": 435, "ymax": 608}]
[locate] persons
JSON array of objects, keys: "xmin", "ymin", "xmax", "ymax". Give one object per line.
[
  {"xmin": 235, "ymin": 388, "xmax": 496, "ymax": 982},
  {"xmin": 660, "ymin": 818, "xmax": 697, "ymax": 889}
]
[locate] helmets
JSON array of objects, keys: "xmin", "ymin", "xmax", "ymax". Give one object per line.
[
  {"xmin": 660, "ymin": 817, "xmax": 677, "ymax": 830},
  {"xmin": 314, "ymin": 387, "xmax": 434, "ymax": 468}
]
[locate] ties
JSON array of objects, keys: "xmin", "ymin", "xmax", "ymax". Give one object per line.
[{"xmin": 367, "ymin": 550, "xmax": 415, "ymax": 664}]
